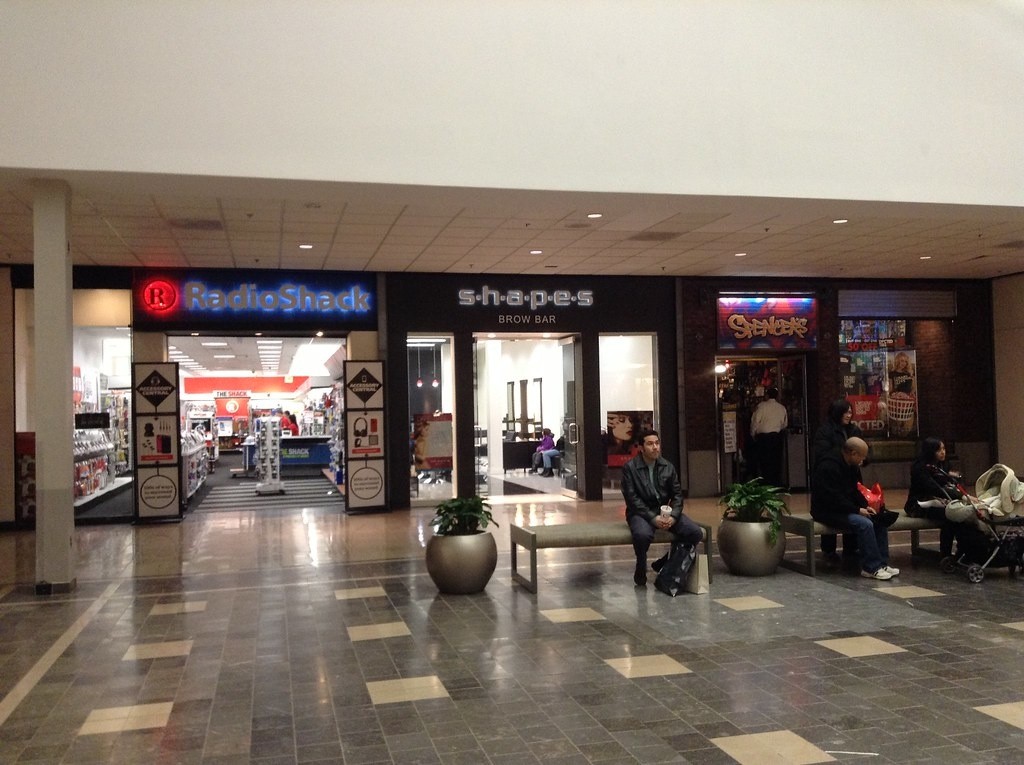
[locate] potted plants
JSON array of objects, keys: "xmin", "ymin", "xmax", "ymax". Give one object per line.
[
  {"xmin": 425, "ymin": 495, "xmax": 499, "ymax": 595},
  {"xmin": 717, "ymin": 477, "xmax": 792, "ymax": 576}
]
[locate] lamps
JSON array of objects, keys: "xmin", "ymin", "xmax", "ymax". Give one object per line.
[
  {"xmin": 431, "ymin": 346, "xmax": 438, "ymax": 387},
  {"xmin": 416, "ymin": 347, "xmax": 422, "ymax": 387}
]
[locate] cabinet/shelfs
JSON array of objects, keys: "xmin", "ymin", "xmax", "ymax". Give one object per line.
[
  {"xmin": 254, "ymin": 417, "xmax": 286, "ymax": 490},
  {"xmin": 181, "ymin": 443, "xmax": 207, "ymax": 500}
]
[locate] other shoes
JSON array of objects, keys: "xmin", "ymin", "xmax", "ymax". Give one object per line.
[
  {"xmin": 540, "ymin": 469, "xmax": 554, "ymax": 477},
  {"xmin": 529, "ymin": 467, "xmax": 537, "ymax": 474},
  {"xmin": 841, "ymin": 550, "xmax": 860, "ymax": 561},
  {"xmin": 822, "ymin": 549, "xmax": 838, "ymax": 561},
  {"xmin": 634, "ymin": 561, "xmax": 648, "ymax": 587}
]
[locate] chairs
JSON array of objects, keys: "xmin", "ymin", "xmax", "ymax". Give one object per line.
[{"xmin": 501, "ymin": 430, "xmax": 516, "ymax": 443}]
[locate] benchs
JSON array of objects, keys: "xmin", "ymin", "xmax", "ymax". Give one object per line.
[
  {"xmin": 533, "ymin": 453, "xmax": 561, "ymax": 476},
  {"xmin": 510, "ymin": 521, "xmax": 713, "ymax": 594},
  {"xmin": 782, "ymin": 508, "xmax": 947, "ymax": 576}
]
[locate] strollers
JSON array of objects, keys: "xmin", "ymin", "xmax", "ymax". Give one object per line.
[{"xmin": 924, "ymin": 462, "xmax": 1024, "ymax": 583}]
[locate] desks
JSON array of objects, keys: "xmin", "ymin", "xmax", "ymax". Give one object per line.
[{"xmin": 479, "ymin": 441, "xmax": 540, "ymax": 474}]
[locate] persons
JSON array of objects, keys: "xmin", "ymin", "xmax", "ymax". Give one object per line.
[
  {"xmin": 809, "ymin": 437, "xmax": 900, "ymax": 581},
  {"xmin": 607, "ymin": 411, "xmax": 639, "ymax": 455},
  {"xmin": 812, "ymin": 399, "xmax": 863, "ymax": 570},
  {"xmin": 888, "ymin": 353, "xmax": 916, "ymax": 397},
  {"xmin": 619, "ymin": 430, "xmax": 703, "ymax": 587},
  {"xmin": 905, "ymin": 439, "xmax": 983, "ymax": 572},
  {"xmin": 527, "ymin": 428, "xmax": 554, "ymax": 474},
  {"xmin": 750, "ymin": 387, "xmax": 788, "ymax": 489},
  {"xmin": 538, "ymin": 433, "xmax": 564, "ymax": 477},
  {"xmin": 280, "ymin": 411, "xmax": 299, "ymax": 435}
]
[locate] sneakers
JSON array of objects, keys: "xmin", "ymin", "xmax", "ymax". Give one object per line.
[
  {"xmin": 882, "ymin": 564, "xmax": 900, "ymax": 576},
  {"xmin": 859, "ymin": 566, "xmax": 893, "ymax": 581}
]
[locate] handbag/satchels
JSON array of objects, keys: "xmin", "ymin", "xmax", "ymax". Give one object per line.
[
  {"xmin": 652, "ymin": 559, "xmax": 663, "ymax": 573},
  {"xmin": 654, "ymin": 540, "xmax": 697, "ymax": 597},
  {"xmin": 684, "ymin": 551, "xmax": 710, "ymax": 594},
  {"xmin": 857, "ymin": 482, "xmax": 885, "ymax": 513}
]
